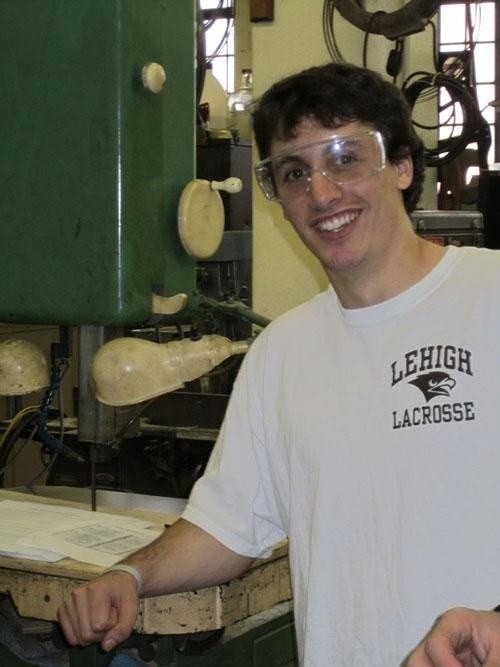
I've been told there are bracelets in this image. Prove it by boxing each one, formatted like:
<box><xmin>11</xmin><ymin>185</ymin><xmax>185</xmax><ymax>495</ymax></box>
<box><xmin>101</xmin><ymin>564</ymin><xmax>143</xmax><ymax>593</ymax></box>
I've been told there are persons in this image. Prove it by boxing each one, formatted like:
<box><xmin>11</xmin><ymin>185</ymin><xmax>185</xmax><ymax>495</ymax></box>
<box><xmin>56</xmin><ymin>61</ymin><xmax>499</xmax><ymax>667</ymax></box>
<box><xmin>400</xmin><ymin>607</ymin><xmax>500</xmax><ymax>667</ymax></box>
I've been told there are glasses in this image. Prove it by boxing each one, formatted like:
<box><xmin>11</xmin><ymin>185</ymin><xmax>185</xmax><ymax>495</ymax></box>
<box><xmin>250</xmin><ymin>127</ymin><xmax>388</xmax><ymax>205</ymax></box>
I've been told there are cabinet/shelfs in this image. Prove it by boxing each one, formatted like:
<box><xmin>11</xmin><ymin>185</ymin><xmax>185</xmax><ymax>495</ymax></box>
<box><xmin>194</xmin><ymin>142</ymin><xmax>255</xmax><ymax>235</ymax></box>
